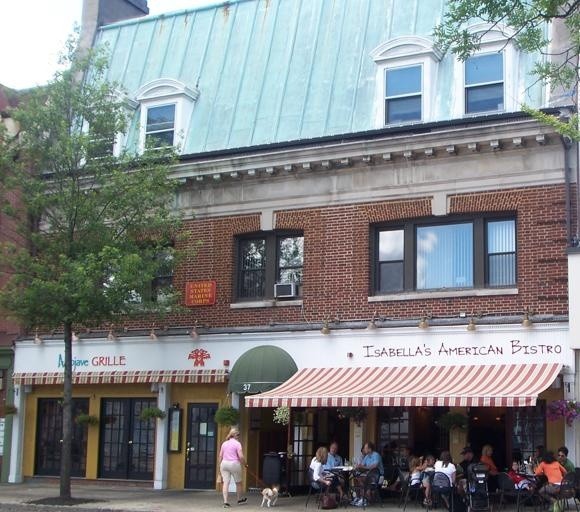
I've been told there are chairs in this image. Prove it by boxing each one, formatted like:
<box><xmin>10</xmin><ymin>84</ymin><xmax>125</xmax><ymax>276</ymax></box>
<box><xmin>303</xmin><ymin>463</ymin><xmax>580</xmax><ymax>511</ymax></box>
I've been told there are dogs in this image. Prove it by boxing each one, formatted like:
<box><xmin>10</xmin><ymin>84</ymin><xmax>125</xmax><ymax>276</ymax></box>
<box><xmin>260</xmin><ymin>483</ymin><xmax>280</xmax><ymax>508</ymax></box>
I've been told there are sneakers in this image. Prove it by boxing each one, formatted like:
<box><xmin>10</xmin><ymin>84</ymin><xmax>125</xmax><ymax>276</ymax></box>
<box><xmin>423</xmin><ymin>498</ymin><xmax>433</xmax><ymax>505</ymax></box>
<box><xmin>224</xmin><ymin>503</ymin><xmax>230</xmax><ymax>507</ymax></box>
<box><xmin>238</xmin><ymin>498</ymin><xmax>247</xmax><ymax>503</ymax></box>
<box><xmin>350</xmin><ymin>497</ymin><xmax>371</xmax><ymax>507</ymax></box>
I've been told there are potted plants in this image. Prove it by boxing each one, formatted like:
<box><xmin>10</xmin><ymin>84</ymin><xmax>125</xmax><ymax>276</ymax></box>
<box><xmin>213</xmin><ymin>407</ymin><xmax>241</xmax><ymax>433</ymax></box>
<box><xmin>139</xmin><ymin>407</ymin><xmax>166</xmax><ymax>428</ymax></box>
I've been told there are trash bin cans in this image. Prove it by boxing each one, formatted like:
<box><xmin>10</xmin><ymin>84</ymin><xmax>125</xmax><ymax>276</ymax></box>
<box><xmin>263</xmin><ymin>454</ymin><xmax>281</xmax><ymax>485</ymax></box>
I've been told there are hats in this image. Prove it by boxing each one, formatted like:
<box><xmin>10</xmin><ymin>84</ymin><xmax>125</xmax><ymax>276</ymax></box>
<box><xmin>460</xmin><ymin>447</ymin><xmax>472</xmax><ymax>455</ymax></box>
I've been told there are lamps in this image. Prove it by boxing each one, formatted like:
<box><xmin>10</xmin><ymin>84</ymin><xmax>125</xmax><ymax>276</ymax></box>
<box><xmin>32</xmin><ymin>319</ymin><xmax>209</xmax><ymax>345</ymax></box>
<box><xmin>320</xmin><ymin>306</ymin><xmax>542</xmax><ymax>335</ymax></box>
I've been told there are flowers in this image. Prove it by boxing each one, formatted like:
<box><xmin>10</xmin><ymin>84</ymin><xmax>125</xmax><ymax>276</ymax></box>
<box><xmin>546</xmin><ymin>397</ymin><xmax>580</xmax><ymax>427</ymax></box>
<box><xmin>273</xmin><ymin>407</ymin><xmax>290</xmax><ymax>427</ymax></box>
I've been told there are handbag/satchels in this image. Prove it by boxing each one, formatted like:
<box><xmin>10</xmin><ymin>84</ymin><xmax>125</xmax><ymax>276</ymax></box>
<box><xmin>320</xmin><ymin>494</ymin><xmax>337</xmax><ymax>509</ymax></box>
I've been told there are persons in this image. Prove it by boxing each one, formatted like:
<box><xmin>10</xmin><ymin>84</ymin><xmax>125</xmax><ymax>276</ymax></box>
<box><xmin>310</xmin><ymin>440</ymin><xmax>576</xmax><ymax>510</ymax></box>
<box><xmin>217</xmin><ymin>426</ymin><xmax>247</xmax><ymax>509</ymax></box>
